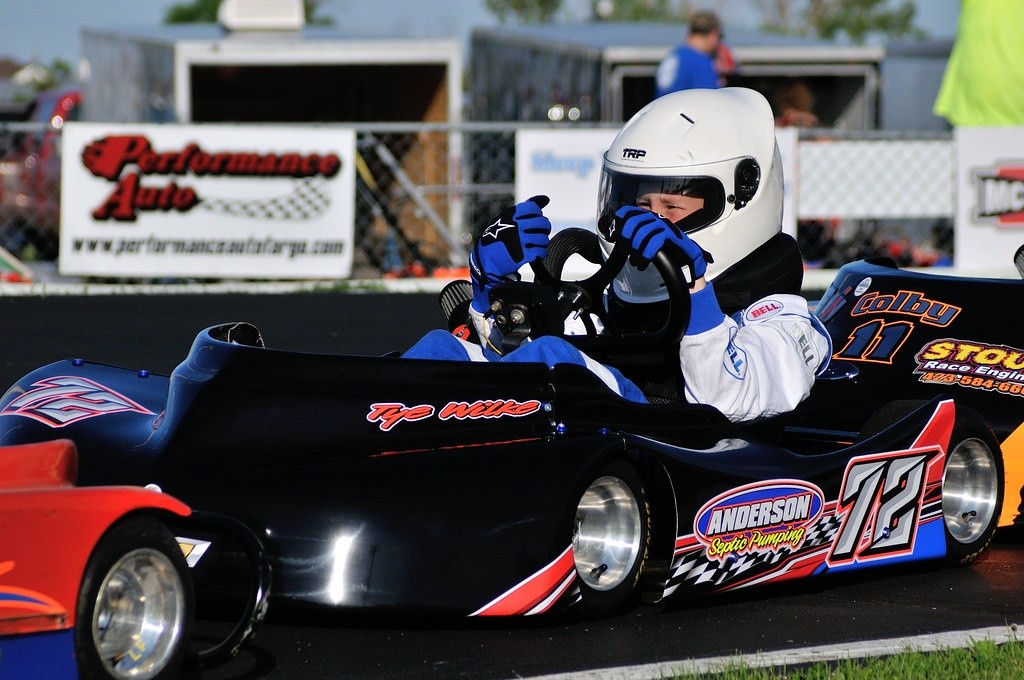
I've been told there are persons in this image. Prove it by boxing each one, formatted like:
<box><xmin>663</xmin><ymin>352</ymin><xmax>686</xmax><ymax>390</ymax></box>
<box><xmin>400</xmin><ymin>85</ymin><xmax>833</xmax><ymax>425</ymax></box>
<box><xmin>770</xmin><ymin>74</ymin><xmax>842</xmax><ymax>266</ymax></box>
<box><xmin>655</xmin><ymin>9</ymin><xmax>725</xmax><ymax>102</ymax></box>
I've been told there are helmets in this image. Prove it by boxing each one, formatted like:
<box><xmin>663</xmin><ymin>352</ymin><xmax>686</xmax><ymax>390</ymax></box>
<box><xmin>595</xmin><ymin>87</ymin><xmax>783</xmax><ymax>306</ymax></box>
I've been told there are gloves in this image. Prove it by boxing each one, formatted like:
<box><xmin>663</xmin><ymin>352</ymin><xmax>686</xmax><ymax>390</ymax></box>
<box><xmin>598</xmin><ymin>206</ymin><xmax>712</xmax><ymax>287</ymax></box>
<box><xmin>467</xmin><ymin>195</ymin><xmax>550</xmax><ymax>312</ymax></box>
